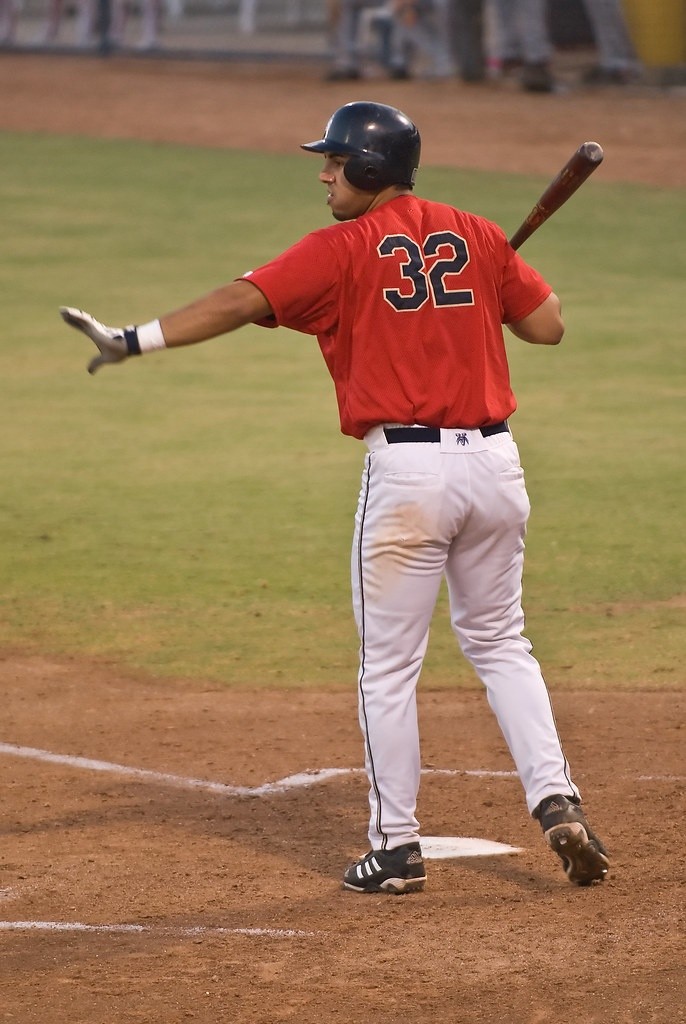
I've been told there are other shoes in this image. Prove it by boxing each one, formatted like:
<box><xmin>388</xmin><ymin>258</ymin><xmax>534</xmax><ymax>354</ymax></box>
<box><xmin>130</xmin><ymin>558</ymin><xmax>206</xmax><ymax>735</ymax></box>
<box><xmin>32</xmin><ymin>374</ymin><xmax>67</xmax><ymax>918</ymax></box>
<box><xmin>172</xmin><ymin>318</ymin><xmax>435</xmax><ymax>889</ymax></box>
<box><xmin>522</xmin><ymin>63</ymin><xmax>553</xmax><ymax>91</ymax></box>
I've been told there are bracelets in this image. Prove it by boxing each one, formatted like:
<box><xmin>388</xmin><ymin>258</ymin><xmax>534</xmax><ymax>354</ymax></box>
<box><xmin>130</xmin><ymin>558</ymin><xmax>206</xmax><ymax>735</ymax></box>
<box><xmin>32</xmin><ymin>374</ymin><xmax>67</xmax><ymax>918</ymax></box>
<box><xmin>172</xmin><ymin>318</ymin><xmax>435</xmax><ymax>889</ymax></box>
<box><xmin>136</xmin><ymin>318</ymin><xmax>166</xmax><ymax>356</ymax></box>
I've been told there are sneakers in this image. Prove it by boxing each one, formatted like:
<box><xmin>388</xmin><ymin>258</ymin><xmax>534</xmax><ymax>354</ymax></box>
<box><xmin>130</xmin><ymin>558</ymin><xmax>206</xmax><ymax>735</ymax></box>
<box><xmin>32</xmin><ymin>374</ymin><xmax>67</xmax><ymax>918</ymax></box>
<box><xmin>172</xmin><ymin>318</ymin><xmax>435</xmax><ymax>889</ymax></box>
<box><xmin>540</xmin><ymin>795</ymin><xmax>610</xmax><ymax>888</ymax></box>
<box><xmin>344</xmin><ymin>841</ymin><xmax>427</xmax><ymax>894</ymax></box>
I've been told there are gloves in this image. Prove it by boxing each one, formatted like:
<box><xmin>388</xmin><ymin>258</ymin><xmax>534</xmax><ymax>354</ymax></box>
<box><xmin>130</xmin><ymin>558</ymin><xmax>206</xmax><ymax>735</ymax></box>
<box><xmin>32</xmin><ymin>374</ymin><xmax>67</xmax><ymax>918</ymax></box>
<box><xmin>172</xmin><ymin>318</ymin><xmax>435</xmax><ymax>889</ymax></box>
<box><xmin>59</xmin><ymin>306</ymin><xmax>166</xmax><ymax>376</ymax></box>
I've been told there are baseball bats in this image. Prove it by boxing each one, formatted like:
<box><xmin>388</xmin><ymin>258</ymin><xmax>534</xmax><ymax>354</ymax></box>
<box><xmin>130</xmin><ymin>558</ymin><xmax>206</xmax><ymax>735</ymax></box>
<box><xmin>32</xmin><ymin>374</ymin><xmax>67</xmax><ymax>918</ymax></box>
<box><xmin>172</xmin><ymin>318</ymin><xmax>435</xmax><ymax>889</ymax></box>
<box><xmin>508</xmin><ymin>139</ymin><xmax>607</xmax><ymax>251</ymax></box>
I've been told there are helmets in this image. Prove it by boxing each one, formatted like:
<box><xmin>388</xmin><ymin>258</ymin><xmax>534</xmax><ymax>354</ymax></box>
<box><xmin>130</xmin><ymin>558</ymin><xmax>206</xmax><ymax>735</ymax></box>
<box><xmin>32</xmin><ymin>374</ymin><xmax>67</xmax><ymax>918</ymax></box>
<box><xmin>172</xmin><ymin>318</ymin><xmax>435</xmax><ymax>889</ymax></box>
<box><xmin>300</xmin><ymin>101</ymin><xmax>421</xmax><ymax>190</ymax></box>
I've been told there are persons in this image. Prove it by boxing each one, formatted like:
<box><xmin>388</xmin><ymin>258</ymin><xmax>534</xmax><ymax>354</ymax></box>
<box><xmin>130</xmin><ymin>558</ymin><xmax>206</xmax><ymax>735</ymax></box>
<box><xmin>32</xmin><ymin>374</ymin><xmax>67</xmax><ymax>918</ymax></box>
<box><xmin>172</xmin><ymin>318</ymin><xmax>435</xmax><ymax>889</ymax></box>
<box><xmin>59</xmin><ymin>100</ymin><xmax>612</xmax><ymax>896</ymax></box>
<box><xmin>322</xmin><ymin>0</ymin><xmax>633</xmax><ymax>102</ymax></box>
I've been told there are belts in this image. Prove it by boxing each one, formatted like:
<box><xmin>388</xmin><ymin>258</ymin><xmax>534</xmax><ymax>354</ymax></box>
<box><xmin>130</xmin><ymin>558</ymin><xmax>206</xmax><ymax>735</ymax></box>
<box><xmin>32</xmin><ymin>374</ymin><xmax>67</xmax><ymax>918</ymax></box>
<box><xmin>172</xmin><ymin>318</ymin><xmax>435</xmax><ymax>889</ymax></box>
<box><xmin>384</xmin><ymin>421</ymin><xmax>510</xmax><ymax>444</ymax></box>
